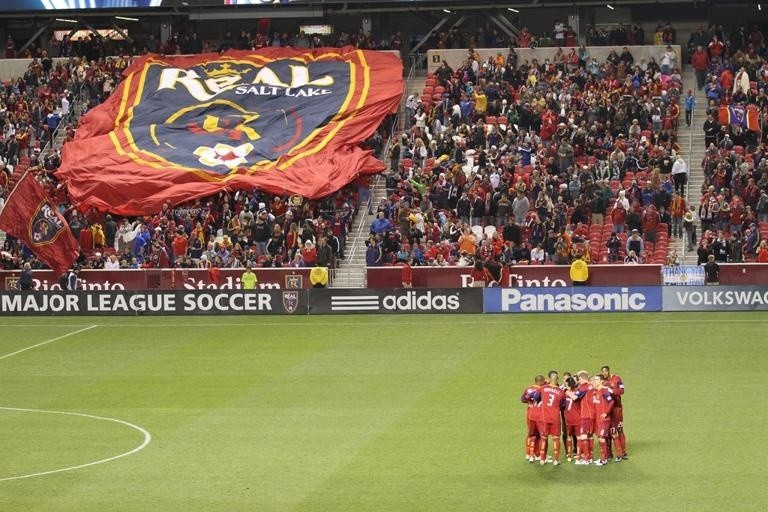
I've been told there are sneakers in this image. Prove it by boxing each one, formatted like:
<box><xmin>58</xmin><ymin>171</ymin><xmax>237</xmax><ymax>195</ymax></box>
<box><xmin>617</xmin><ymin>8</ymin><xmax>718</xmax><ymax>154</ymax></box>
<box><xmin>526</xmin><ymin>454</ymin><xmax>560</xmax><ymax>466</ymax></box>
<box><xmin>594</xmin><ymin>459</ymin><xmax>608</xmax><ymax>466</ymax></box>
<box><xmin>616</xmin><ymin>454</ymin><xmax>628</xmax><ymax>462</ymax></box>
<box><xmin>566</xmin><ymin>453</ymin><xmax>592</xmax><ymax>465</ymax></box>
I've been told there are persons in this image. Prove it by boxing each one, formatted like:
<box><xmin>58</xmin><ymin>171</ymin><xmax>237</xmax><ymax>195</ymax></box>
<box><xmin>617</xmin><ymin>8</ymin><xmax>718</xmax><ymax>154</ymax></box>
<box><xmin>523</xmin><ymin>366</ymin><xmax>628</xmax><ymax>466</ymax></box>
<box><xmin>0</xmin><ymin>22</ymin><xmax>768</xmax><ymax>291</ymax></box>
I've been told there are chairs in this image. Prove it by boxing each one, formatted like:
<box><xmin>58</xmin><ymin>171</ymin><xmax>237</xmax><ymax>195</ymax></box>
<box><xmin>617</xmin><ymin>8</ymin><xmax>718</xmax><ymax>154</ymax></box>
<box><xmin>383</xmin><ymin>71</ymin><xmax>668</xmax><ymax>266</ymax></box>
<box><xmin>703</xmin><ymin>82</ymin><xmax>767</xmax><ymax>263</ymax></box>
<box><xmin>6</xmin><ymin>138</ymin><xmax>41</xmax><ymax>192</ymax></box>
